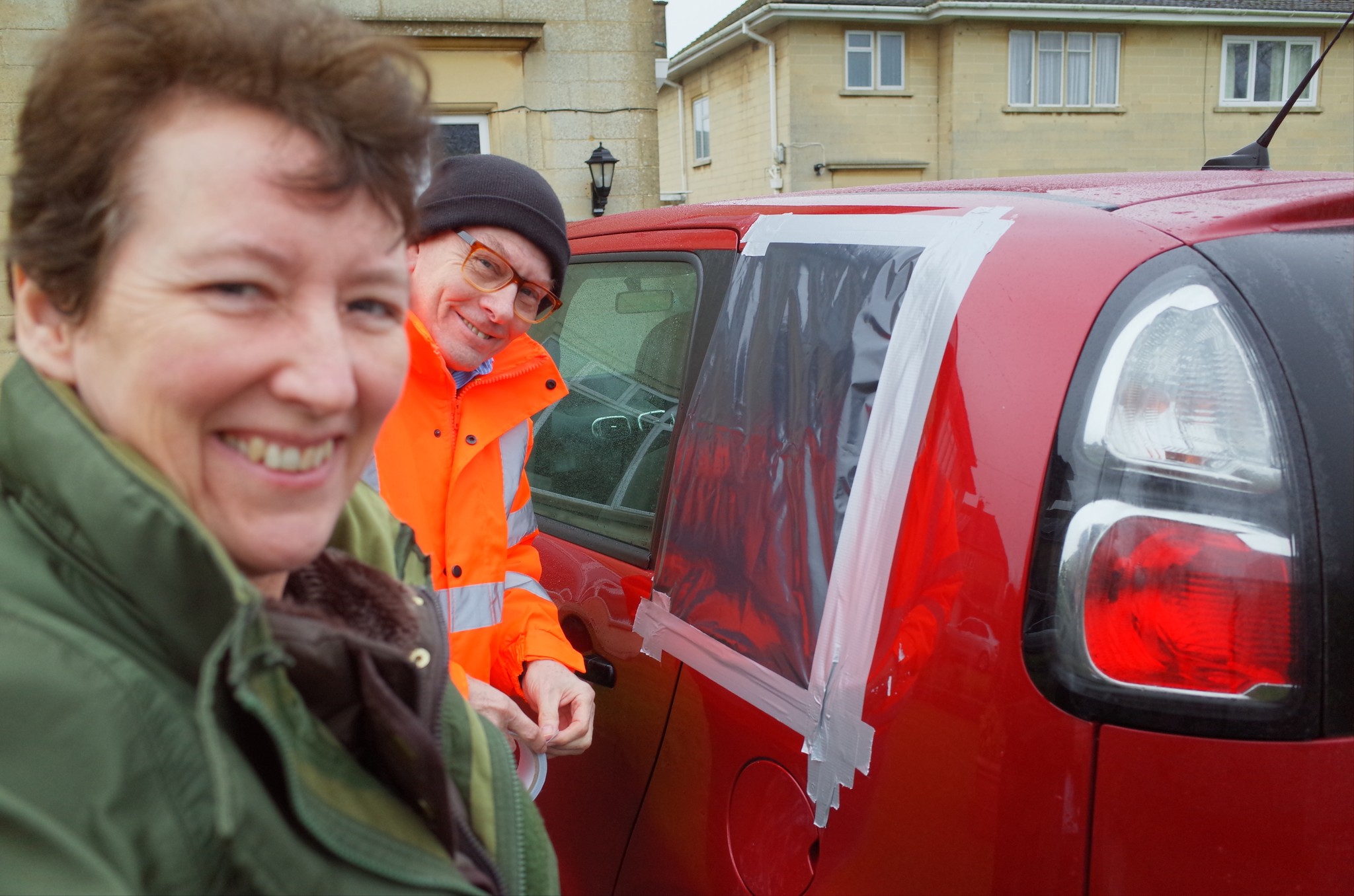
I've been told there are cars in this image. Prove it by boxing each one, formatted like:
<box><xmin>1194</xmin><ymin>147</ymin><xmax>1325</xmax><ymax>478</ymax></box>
<box><xmin>524</xmin><ymin>11</ymin><xmax>1354</xmax><ymax>895</ymax></box>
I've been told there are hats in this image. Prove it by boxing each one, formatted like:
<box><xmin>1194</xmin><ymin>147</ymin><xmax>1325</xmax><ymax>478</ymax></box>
<box><xmin>407</xmin><ymin>154</ymin><xmax>571</xmax><ymax>315</ymax></box>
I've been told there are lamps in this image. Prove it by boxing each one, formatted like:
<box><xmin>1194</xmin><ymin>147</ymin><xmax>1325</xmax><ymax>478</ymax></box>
<box><xmin>583</xmin><ymin>141</ymin><xmax>621</xmax><ymax>218</ymax></box>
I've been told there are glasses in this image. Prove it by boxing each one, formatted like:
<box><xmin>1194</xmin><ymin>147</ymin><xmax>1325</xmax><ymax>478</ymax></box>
<box><xmin>450</xmin><ymin>226</ymin><xmax>563</xmax><ymax>325</ymax></box>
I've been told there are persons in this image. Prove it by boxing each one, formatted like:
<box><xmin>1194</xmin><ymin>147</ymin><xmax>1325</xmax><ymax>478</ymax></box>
<box><xmin>354</xmin><ymin>152</ymin><xmax>598</xmax><ymax>766</ymax></box>
<box><xmin>0</xmin><ymin>0</ymin><xmax>564</xmax><ymax>896</ymax></box>
